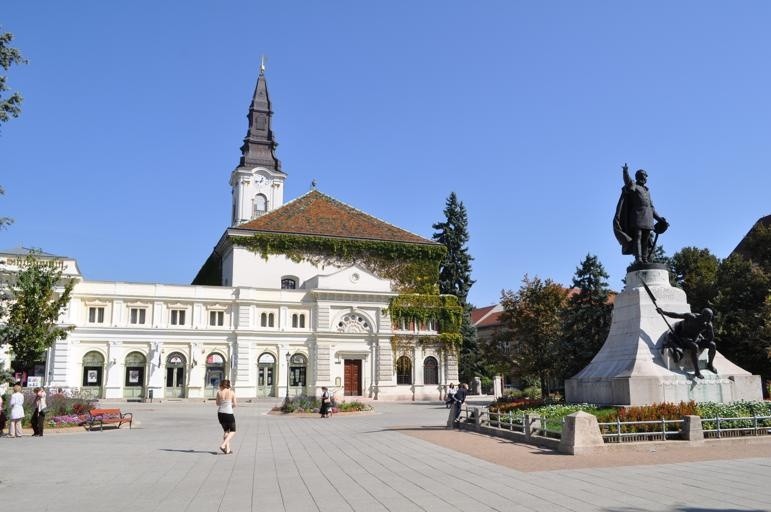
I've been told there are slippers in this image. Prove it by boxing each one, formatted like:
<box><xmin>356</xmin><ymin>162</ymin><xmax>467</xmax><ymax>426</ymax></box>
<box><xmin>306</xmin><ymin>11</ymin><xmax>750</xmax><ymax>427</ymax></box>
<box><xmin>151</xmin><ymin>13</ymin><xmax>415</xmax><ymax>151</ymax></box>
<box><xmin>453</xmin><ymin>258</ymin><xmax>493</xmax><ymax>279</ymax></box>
<box><xmin>220</xmin><ymin>445</ymin><xmax>234</xmax><ymax>454</ymax></box>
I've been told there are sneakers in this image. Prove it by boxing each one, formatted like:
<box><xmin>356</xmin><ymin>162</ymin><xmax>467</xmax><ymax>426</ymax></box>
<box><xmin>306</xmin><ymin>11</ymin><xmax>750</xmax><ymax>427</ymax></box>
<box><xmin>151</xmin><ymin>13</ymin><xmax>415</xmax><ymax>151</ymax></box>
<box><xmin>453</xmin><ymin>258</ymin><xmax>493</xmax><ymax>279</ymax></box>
<box><xmin>446</xmin><ymin>405</ymin><xmax>454</xmax><ymax>408</ymax></box>
<box><xmin>321</xmin><ymin>415</ymin><xmax>330</xmax><ymax>418</ymax></box>
<box><xmin>454</xmin><ymin>418</ymin><xmax>461</xmax><ymax>423</ymax></box>
<box><xmin>0</xmin><ymin>431</ymin><xmax>44</xmax><ymax>438</ymax></box>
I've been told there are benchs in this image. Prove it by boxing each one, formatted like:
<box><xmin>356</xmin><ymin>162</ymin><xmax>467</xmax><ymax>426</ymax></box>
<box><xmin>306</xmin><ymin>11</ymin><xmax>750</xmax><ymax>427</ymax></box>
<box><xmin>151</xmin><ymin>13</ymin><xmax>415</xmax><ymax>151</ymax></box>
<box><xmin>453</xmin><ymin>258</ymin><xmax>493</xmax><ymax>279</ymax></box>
<box><xmin>88</xmin><ymin>407</ymin><xmax>133</xmax><ymax>432</ymax></box>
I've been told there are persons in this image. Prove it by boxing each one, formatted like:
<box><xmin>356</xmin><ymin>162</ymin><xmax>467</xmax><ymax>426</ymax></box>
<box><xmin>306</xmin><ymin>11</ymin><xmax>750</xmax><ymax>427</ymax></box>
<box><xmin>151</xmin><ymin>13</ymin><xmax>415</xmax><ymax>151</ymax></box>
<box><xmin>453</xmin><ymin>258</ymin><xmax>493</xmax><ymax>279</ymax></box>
<box><xmin>656</xmin><ymin>307</ymin><xmax>718</xmax><ymax>379</ymax></box>
<box><xmin>0</xmin><ymin>397</ymin><xmax>7</xmax><ymax>436</ymax></box>
<box><xmin>320</xmin><ymin>386</ymin><xmax>338</xmax><ymax>417</ymax></box>
<box><xmin>445</xmin><ymin>383</ymin><xmax>455</xmax><ymax>408</ymax></box>
<box><xmin>6</xmin><ymin>385</ymin><xmax>24</xmax><ymax>438</ymax></box>
<box><xmin>31</xmin><ymin>387</ymin><xmax>47</xmax><ymax>438</ymax></box>
<box><xmin>54</xmin><ymin>388</ymin><xmax>65</xmax><ymax>396</ymax></box>
<box><xmin>612</xmin><ymin>162</ymin><xmax>670</xmax><ymax>263</ymax></box>
<box><xmin>454</xmin><ymin>383</ymin><xmax>469</xmax><ymax>423</ymax></box>
<box><xmin>216</xmin><ymin>379</ymin><xmax>237</xmax><ymax>454</ymax></box>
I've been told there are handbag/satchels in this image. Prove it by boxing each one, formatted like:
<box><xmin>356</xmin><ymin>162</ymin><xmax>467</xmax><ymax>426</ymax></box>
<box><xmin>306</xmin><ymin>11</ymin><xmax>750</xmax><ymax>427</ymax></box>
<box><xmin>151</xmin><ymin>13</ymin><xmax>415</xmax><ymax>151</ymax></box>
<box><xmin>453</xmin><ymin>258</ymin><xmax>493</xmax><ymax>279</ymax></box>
<box><xmin>325</xmin><ymin>402</ymin><xmax>334</xmax><ymax>407</ymax></box>
